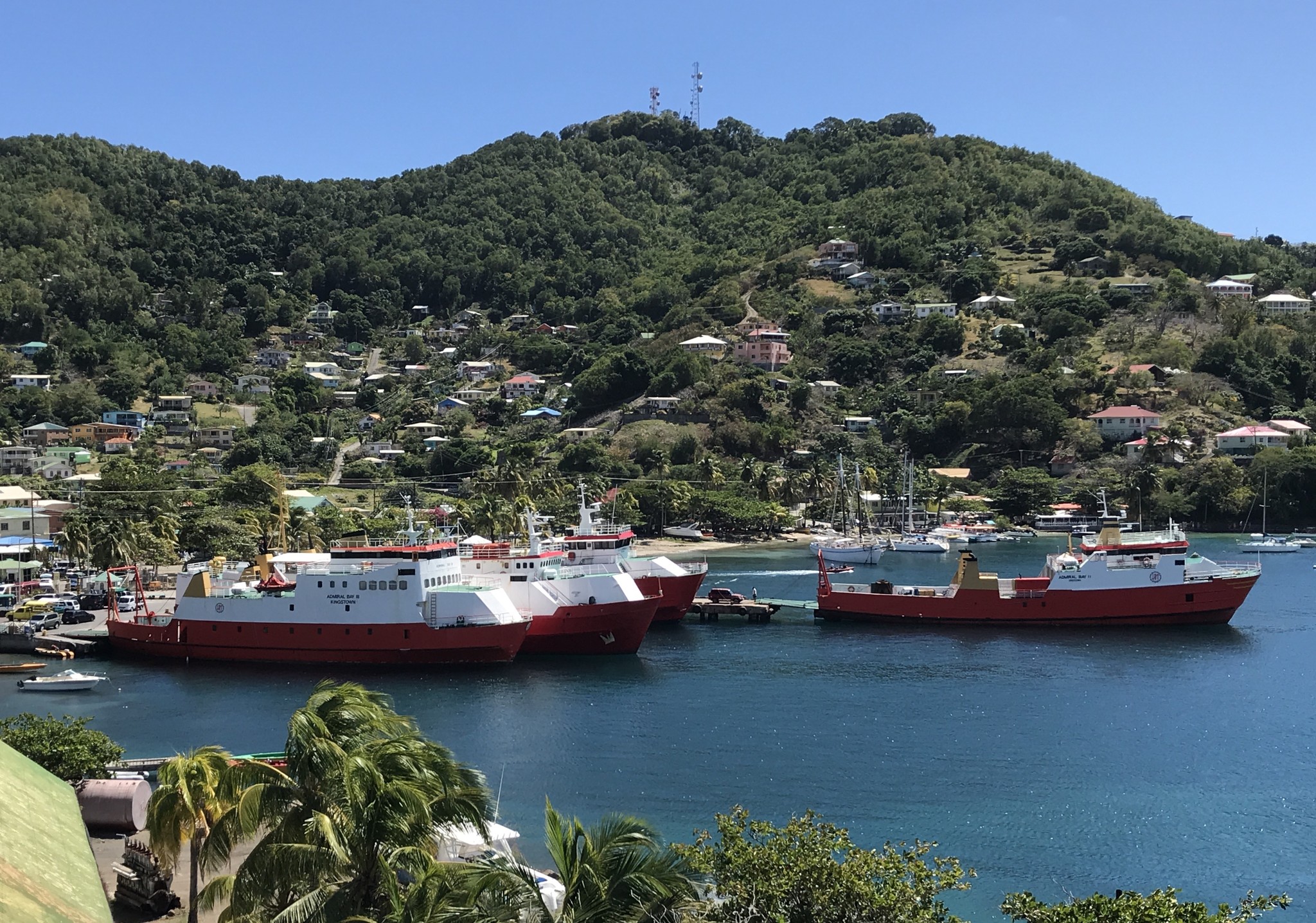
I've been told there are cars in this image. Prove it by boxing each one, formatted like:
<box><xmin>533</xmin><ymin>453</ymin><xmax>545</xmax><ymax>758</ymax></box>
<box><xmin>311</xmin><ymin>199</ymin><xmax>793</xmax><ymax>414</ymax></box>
<box><xmin>0</xmin><ymin>558</ymin><xmax>130</xmax><ymax>618</ymax></box>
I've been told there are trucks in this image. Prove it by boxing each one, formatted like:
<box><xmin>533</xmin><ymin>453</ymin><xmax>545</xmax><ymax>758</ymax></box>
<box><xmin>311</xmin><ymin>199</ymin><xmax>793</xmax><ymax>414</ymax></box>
<box><xmin>117</xmin><ymin>595</ymin><xmax>144</xmax><ymax>612</ymax></box>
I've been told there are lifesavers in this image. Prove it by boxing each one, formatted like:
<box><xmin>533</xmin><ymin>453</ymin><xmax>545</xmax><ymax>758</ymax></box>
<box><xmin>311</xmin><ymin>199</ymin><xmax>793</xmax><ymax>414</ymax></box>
<box><xmin>848</xmin><ymin>586</ymin><xmax>855</xmax><ymax>592</ymax></box>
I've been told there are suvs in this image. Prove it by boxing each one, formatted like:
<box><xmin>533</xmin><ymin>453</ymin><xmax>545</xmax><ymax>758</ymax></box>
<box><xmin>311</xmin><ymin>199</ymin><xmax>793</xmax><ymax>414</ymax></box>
<box><xmin>29</xmin><ymin>612</ymin><xmax>61</xmax><ymax>632</ymax></box>
<box><xmin>708</xmin><ymin>588</ymin><xmax>744</xmax><ymax>604</ymax></box>
<box><xmin>54</xmin><ymin>599</ymin><xmax>80</xmax><ymax>614</ymax></box>
<box><xmin>61</xmin><ymin>610</ymin><xmax>95</xmax><ymax>624</ymax></box>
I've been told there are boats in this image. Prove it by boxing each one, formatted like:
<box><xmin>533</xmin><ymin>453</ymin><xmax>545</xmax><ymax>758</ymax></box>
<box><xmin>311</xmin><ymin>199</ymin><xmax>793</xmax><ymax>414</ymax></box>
<box><xmin>253</xmin><ymin>574</ymin><xmax>296</xmax><ymax>593</ymax></box>
<box><xmin>226</xmin><ymin>751</ymin><xmax>289</xmax><ymax>776</ymax></box>
<box><xmin>0</xmin><ymin>663</ymin><xmax>48</xmax><ymax>673</ymax></box>
<box><xmin>1235</xmin><ymin>467</ymin><xmax>1316</xmax><ymax>551</ymax></box>
<box><xmin>662</xmin><ymin>521</ymin><xmax>718</xmax><ymax>540</ymax></box>
<box><xmin>104</xmin><ymin>473</ymin><xmax>709</xmax><ymax>668</ymax></box>
<box><xmin>1070</xmin><ymin>488</ymin><xmax>1190</xmax><ymax>556</ymax></box>
<box><xmin>433</xmin><ymin>762</ymin><xmax>566</xmax><ymax>923</ymax></box>
<box><xmin>813</xmin><ymin>547</ymin><xmax>1263</xmax><ymax>626</ymax></box>
<box><xmin>17</xmin><ymin>669</ymin><xmax>106</xmax><ymax>691</ymax></box>
<box><xmin>809</xmin><ymin>448</ymin><xmax>1038</xmax><ymax>564</ymax></box>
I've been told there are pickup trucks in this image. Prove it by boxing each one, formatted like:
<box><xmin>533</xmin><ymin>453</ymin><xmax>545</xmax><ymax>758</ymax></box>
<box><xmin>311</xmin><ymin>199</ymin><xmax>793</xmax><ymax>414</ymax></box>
<box><xmin>5</xmin><ymin>606</ymin><xmax>56</xmax><ymax>621</ymax></box>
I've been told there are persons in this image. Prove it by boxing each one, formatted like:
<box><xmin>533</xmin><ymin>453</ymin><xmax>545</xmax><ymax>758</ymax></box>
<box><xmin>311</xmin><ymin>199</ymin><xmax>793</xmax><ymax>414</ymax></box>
<box><xmin>830</xmin><ymin>561</ymin><xmax>834</xmax><ymax>569</ymax></box>
<box><xmin>752</xmin><ymin>586</ymin><xmax>757</xmax><ymax>602</ymax></box>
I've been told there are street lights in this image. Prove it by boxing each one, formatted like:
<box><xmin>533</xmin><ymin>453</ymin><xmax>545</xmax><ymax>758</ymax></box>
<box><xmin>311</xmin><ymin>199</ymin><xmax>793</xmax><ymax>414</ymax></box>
<box><xmin>19</xmin><ymin>533</ymin><xmax>41</xmax><ymax>605</ymax></box>
